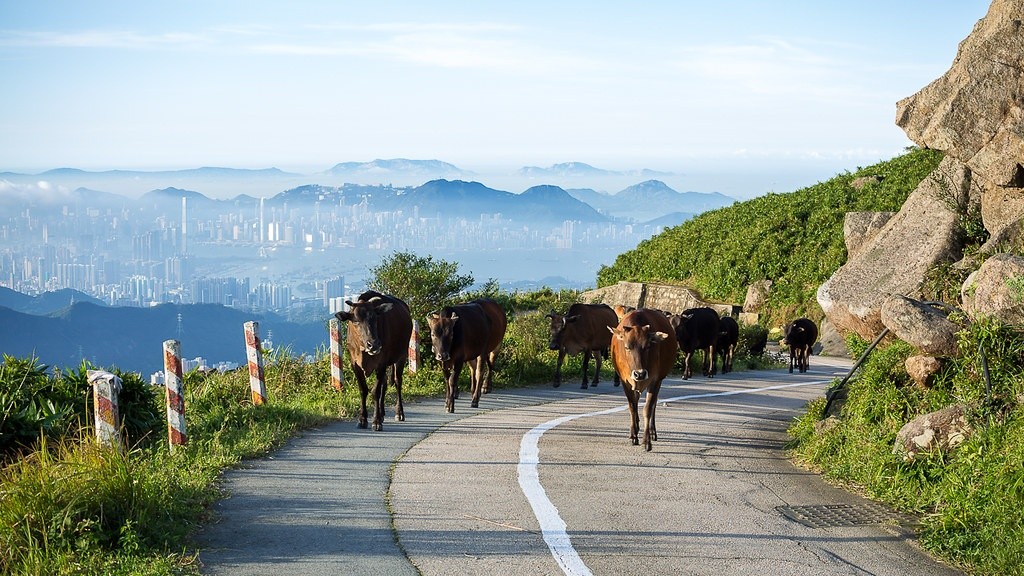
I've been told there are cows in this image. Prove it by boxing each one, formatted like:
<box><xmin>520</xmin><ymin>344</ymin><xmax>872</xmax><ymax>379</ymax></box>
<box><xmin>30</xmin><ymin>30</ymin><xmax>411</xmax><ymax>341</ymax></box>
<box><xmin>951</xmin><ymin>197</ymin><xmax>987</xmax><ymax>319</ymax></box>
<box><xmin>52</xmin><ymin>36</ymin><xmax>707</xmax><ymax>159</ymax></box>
<box><xmin>663</xmin><ymin>307</ymin><xmax>739</xmax><ymax>381</ymax></box>
<box><xmin>783</xmin><ymin>318</ymin><xmax>819</xmax><ymax>374</ymax></box>
<box><xmin>544</xmin><ymin>303</ymin><xmax>619</xmax><ymax>389</ymax></box>
<box><xmin>425</xmin><ymin>298</ymin><xmax>507</xmax><ymax>413</ymax></box>
<box><xmin>334</xmin><ymin>291</ymin><xmax>413</xmax><ymax>431</ymax></box>
<box><xmin>611</xmin><ymin>308</ymin><xmax>677</xmax><ymax>451</ymax></box>
